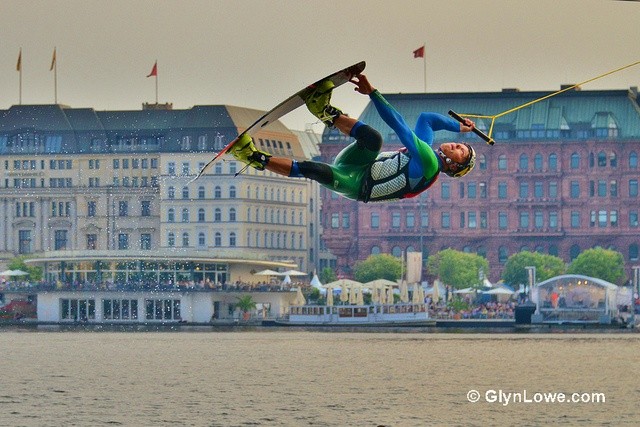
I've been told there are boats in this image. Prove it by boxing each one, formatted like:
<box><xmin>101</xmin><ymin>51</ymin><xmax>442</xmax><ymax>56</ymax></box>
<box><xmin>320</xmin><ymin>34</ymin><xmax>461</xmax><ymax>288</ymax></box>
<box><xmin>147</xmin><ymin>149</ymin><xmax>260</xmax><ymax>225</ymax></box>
<box><xmin>274</xmin><ymin>302</ymin><xmax>437</xmax><ymax>326</ymax></box>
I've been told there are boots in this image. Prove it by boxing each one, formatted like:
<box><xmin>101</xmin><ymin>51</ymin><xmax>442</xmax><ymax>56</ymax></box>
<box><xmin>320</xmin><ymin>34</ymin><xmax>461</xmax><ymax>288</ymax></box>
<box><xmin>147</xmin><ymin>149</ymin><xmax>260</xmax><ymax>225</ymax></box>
<box><xmin>305</xmin><ymin>80</ymin><xmax>348</xmax><ymax>130</ymax></box>
<box><xmin>231</xmin><ymin>134</ymin><xmax>271</xmax><ymax>171</ymax></box>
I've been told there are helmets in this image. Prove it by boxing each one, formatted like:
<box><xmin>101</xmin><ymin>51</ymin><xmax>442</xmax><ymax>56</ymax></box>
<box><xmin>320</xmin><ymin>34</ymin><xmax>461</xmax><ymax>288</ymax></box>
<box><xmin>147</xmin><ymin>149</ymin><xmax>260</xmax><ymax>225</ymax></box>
<box><xmin>447</xmin><ymin>142</ymin><xmax>476</xmax><ymax>178</ymax></box>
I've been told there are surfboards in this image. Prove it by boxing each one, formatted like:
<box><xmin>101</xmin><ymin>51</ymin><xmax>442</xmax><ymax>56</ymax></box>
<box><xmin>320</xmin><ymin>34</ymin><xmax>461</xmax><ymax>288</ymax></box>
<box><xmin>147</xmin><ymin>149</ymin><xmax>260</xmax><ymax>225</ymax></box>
<box><xmin>193</xmin><ymin>61</ymin><xmax>366</xmax><ymax>180</ymax></box>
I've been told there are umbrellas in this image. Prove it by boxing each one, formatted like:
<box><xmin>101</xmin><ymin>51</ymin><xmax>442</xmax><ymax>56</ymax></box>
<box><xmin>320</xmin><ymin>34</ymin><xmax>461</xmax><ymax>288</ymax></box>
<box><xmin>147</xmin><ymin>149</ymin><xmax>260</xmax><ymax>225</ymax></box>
<box><xmin>1</xmin><ymin>268</ymin><xmax>30</xmax><ymax>276</ymax></box>
<box><xmin>279</xmin><ymin>269</ymin><xmax>309</xmax><ymax>276</ymax></box>
<box><xmin>254</xmin><ymin>268</ymin><xmax>283</xmax><ymax>276</ymax></box>
<box><xmin>290</xmin><ymin>277</ymin><xmax>440</xmax><ymax>305</ymax></box>
<box><xmin>481</xmin><ymin>286</ymin><xmax>516</xmax><ymax>295</ymax></box>
<box><xmin>452</xmin><ymin>285</ymin><xmax>487</xmax><ymax>294</ymax></box>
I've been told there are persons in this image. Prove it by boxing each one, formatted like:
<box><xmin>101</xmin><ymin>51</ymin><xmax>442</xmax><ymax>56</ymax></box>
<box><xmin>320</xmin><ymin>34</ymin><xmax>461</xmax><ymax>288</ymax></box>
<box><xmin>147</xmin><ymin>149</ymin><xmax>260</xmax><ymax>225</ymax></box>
<box><xmin>225</xmin><ymin>71</ymin><xmax>477</xmax><ymax>204</ymax></box>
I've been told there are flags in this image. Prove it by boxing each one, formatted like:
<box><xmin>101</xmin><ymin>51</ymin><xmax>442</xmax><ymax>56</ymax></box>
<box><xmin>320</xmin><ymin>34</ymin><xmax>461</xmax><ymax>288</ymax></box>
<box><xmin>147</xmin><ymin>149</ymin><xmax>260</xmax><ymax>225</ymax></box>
<box><xmin>147</xmin><ymin>59</ymin><xmax>158</xmax><ymax>78</ymax></box>
<box><xmin>50</xmin><ymin>46</ymin><xmax>57</xmax><ymax>71</ymax></box>
<box><xmin>414</xmin><ymin>43</ymin><xmax>426</xmax><ymax>58</ymax></box>
<box><xmin>16</xmin><ymin>45</ymin><xmax>22</xmax><ymax>71</ymax></box>
